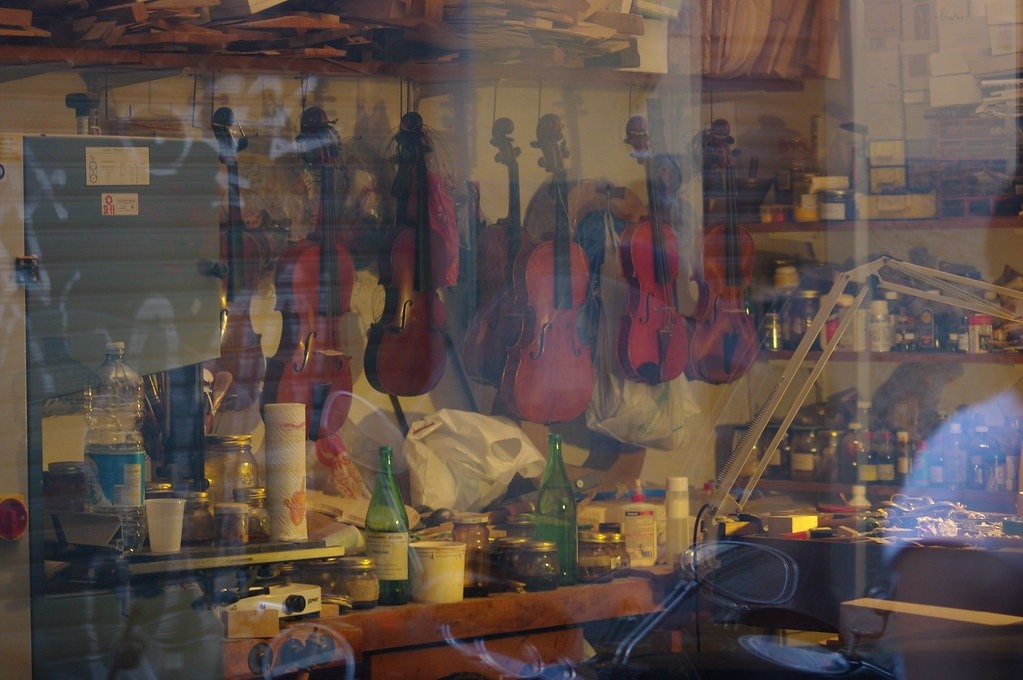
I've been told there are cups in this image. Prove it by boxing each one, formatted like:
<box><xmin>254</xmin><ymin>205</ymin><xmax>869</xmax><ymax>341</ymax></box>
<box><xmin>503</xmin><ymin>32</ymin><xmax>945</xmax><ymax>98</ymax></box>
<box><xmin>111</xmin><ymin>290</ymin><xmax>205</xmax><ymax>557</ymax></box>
<box><xmin>410</xmin><ymin>541</ymin><xmax>467</xmax><ymax>602</ymax></box>
<box><xmin>144</xmin><ymin>498</ymin><xmax>187</xmax><ymax>553</ymax></box>
<box><xmin>43</xmin><ymin>461</ymin><xmax>84</xmax><ymax>504</ymax></box>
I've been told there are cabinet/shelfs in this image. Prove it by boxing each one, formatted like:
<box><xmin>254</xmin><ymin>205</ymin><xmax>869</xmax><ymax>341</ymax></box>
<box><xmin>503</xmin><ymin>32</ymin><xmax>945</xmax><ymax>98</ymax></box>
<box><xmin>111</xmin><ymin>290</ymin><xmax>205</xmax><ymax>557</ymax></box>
<box><xmin>717</xmin><ymin>215</ymin><xmax>1023</xmax><ymax>515</ymax></box>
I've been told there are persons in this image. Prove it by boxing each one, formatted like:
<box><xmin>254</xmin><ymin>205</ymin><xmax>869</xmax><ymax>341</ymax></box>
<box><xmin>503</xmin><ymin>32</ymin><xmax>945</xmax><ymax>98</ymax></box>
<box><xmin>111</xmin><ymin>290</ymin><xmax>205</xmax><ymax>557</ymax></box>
<box><xmin>875</xmin><ymin>157</ymin><xmax>1022</xmax><ymax>680</ymax></box>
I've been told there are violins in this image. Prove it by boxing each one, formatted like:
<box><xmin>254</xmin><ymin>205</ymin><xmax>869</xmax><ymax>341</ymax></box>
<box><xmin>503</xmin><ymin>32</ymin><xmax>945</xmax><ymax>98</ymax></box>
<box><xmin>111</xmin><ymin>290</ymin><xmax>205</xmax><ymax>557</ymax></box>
<box><xmin>364</xmin><ymin>110</ymin><xmax>450</xmax><ymax>399</ymax></box>
<box><xmin>461</xmin><ymin>117</ymin><xmax>533</xmax><ymax>389</ymax></box>
<box><xmin>496</xmin><ymin>114</ymin><xmax>598</xmax><ymax>424</ymax></box>
<box><xmin>685</xmin><ymin>118</ymin><xmax>758</xmax><ymax>386</ymax></box>
<box><xmin>616</xmin><ymin>114</ymin><xmax>691</xmax><ymax>385</ymax></box>
<box><xmin>259</xmin><ymin>104</ymin><xmax>356</xmax><ymax>442</ymax></box>
<box><xmin>190</xmin><ymin>102</ymin><xmax>273</xmax><ymax>413</ymax></box>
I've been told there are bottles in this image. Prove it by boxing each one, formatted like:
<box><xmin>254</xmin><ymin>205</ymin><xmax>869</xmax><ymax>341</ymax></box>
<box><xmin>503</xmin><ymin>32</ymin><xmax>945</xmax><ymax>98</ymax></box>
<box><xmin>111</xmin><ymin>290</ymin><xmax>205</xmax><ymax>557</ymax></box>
<box><xmin>84</xmin><ymin>343</ymin><xmax>144</xmax><ymax>551</ymax></box>
<box><xmin>576</xmin><ymin>482</ymin><xmax>657</xmax><ymax>582</ymax></box>
<box><xmin>452</xmin><ymin>511</ymin><xmax>559</xmax><ymax>596</ymax></box>
<box><xmin>665</xmin><ymin>476</ymin><xmax>688</xmax><ymax>559</ymax></box>
<box><xmin>181</xmin><ymin>430</ymin><xmax>269</xmax><ymax>546</ymax></box>
<box><xmin>845</xmin><ymin>484</ymin><xmax>872</xmax><ymax>507</ymax></box>
<box><xmin>535</xmin><ymin>436</ymin><xmax>577</xmax><ymax>585</ymax></box>
<box><xmin>743</xmin><ymin>133</ymin><xmax>855</xmax><ymax>220</ymax></box>
<box><xmin>761</xmin><ymin>265</ymin><xmax>969</xmax><ymax>353</ymax></box>
<box><xmin>929</xmin><ymin>402</ymin><xmax>1023</xmax><ymax>489</ymax></box>
<box><xmin>279</xmin><ymin>448</ymin><xmax>409</xmax><ymax>610</ymax></box>
<box><xmin>734</xmin><ymin>401</ymin><xmax>929</xmax><ymax>487</ymax></box>
